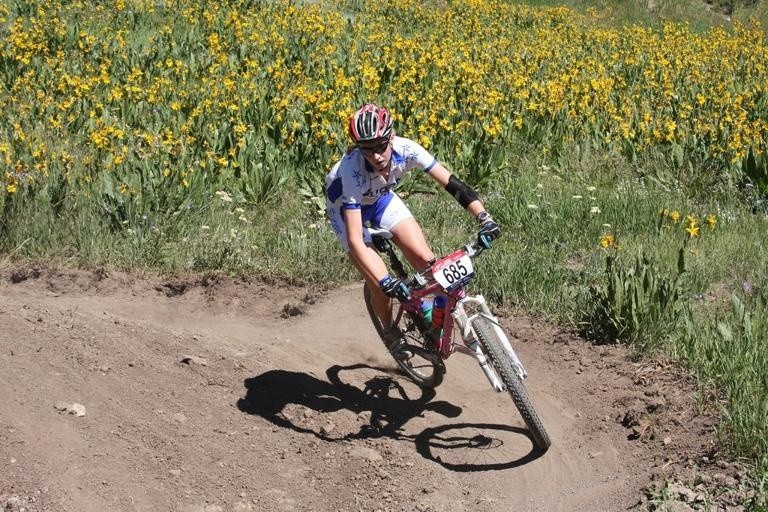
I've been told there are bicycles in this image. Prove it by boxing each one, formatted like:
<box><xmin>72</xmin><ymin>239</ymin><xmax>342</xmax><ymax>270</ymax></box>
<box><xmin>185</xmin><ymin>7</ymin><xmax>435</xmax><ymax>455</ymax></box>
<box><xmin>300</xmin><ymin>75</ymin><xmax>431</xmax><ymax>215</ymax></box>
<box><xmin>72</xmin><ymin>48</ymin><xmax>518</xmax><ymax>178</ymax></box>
<box><xmin>362</xmin><ymin>227</ymin><xmax>551</xmax><ymax>450</ymax></box>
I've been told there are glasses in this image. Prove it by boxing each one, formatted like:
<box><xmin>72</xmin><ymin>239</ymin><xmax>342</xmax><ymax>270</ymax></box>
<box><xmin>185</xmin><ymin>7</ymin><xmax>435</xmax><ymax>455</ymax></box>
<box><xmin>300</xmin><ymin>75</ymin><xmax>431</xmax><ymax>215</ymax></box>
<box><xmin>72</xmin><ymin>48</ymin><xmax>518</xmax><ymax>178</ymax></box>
<box><xmin>357</xmin><ymin>137</ymin><xmax>391</xmax><ymax>157</ymax></box>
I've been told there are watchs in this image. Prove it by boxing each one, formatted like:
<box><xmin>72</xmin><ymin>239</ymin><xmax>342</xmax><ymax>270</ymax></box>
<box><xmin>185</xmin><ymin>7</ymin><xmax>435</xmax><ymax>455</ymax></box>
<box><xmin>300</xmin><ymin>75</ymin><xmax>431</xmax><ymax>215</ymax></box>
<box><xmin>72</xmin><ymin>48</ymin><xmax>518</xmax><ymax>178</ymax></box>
<box><xmin>477</xmin><ymin>211</ymin><xmax>490</xmax><ymax>223</ymax></box>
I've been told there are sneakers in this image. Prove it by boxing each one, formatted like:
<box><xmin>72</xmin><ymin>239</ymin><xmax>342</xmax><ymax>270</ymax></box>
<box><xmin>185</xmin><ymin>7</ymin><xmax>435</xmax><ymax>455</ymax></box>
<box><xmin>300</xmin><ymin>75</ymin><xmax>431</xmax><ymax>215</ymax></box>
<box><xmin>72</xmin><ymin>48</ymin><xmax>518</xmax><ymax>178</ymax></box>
<box><xmin>383</xmin><ymin>320</ymin><xmax>413</xmax><ymax>360</ymax></box>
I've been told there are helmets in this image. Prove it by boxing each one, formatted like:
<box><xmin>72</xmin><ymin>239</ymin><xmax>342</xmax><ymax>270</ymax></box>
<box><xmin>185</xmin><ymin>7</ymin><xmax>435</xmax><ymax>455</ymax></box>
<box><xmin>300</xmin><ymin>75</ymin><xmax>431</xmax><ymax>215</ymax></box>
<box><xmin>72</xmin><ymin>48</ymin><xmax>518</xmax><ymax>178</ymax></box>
<box><xmin>349</xmin><ymin>103</ymin><xmax>394</xmax><ymax>144</ymax></box>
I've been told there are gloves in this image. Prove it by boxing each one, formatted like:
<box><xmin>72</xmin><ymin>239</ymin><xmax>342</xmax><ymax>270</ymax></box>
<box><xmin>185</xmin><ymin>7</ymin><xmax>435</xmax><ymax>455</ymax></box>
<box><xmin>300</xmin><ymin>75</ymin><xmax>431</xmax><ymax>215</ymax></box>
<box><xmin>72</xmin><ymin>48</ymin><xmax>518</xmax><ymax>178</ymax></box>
<box><xmin>380</xmin><ymin>274</ymin><xmax>410</xmax><ymax>303</ymax></box>
<box><xmin>476</xmin><ymin>211</ymin><xmax>502</xmax><ymax>249</ymax></box>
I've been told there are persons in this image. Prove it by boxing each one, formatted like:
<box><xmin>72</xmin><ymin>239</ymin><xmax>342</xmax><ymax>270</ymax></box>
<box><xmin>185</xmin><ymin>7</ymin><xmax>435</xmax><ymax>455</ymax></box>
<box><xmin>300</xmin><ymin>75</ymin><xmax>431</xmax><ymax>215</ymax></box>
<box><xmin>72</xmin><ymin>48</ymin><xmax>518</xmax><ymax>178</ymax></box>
<box><xmin>325</xmin><ymin>103</ymin><xmax>502</xmax><ymax>360</ymax></box>
